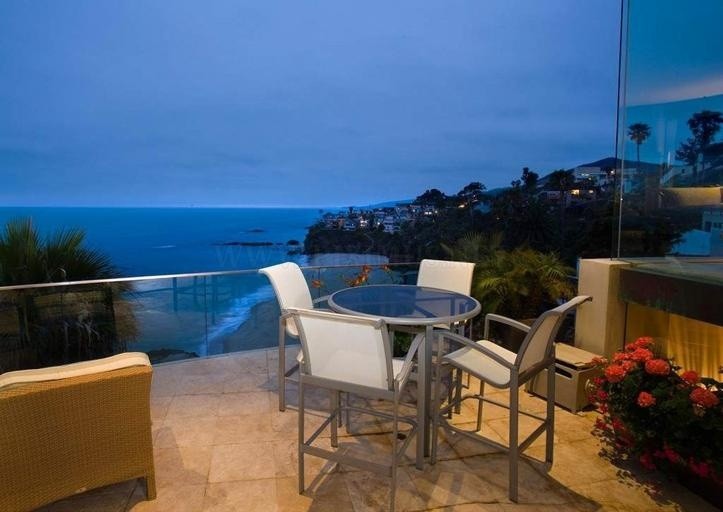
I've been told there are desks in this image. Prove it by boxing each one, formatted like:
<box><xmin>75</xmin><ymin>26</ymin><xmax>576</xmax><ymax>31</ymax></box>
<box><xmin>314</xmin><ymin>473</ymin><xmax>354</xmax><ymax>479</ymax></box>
<box><xmin>328</xmin><ymin>283</ymin><xmax>484</xmax><ymax>463</ymax></box>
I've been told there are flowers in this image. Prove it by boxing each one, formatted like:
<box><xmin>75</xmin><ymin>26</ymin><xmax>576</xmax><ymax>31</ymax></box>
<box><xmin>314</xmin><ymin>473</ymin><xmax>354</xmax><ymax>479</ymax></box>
<box><xmin>584</xmin><ymin>335</ymin><xmax>723</xmax><ymax>496</ymax></box>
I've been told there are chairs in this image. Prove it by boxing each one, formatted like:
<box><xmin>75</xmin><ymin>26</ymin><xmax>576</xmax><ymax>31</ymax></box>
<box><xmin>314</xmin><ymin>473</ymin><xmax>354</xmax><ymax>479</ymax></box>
<box><xmin>397</xmin><ymin>258</ymin><xmax>478</xmax><ymax>418</ymax></box>
<box><xmin>258</xmin><ymin>260</ymin><xmax>351</xmax><ymax>434</ymax></box>
<box><xmin>434</xmin><ymin>292</ymin><xmax>591</xmax><ymax>501</ymax></box>
<box><xmin>287</xmin><ymin>306</ymin><xmax>431</xmax><ymax>509</ymax></box>
<box><xmin>0</xmin><ymin>351</ymin><xmax>157</xmax><ymax>512</ymax></box>
